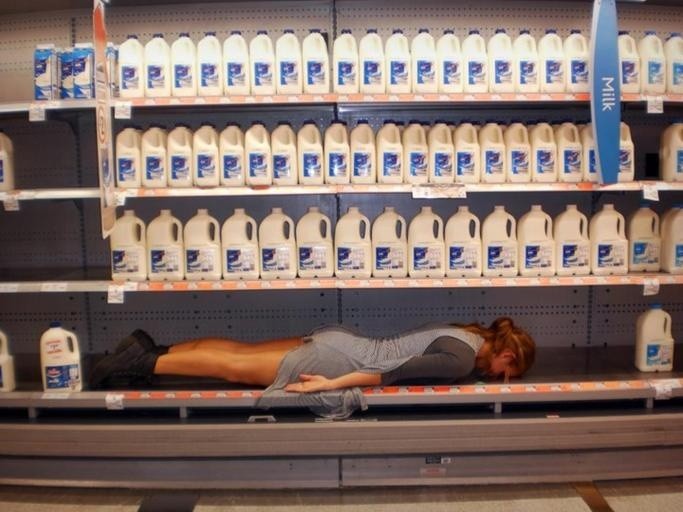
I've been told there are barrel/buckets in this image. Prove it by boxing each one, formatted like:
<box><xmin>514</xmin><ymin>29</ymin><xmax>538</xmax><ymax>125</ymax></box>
<box><xmin>146</xmin><ymin>211</ymin><xmax>183</xmax><ymax>280</ymax></box>
<box><xmin>221</xmin><ymin>208</ymin><xmax>259</xmax><ymax>280</ymax></box>
<box><xmin>302</xmin><ymin>29</ymin><xmax>330</xmax><ymax>93</ymax></box>
<box><xmin>192</xmin><ymin>122</ymin><xmax>219</xmax><ymax>186</ymax></box>
<box><xmin>526</xmin><ymin>118</ymin><xmax>537</xmax><ymax>132</ymax></box>
<box><xmin>272</xmin><ymin>121</ymin><xmax>298</xmax><ymax>186</ymax></box>
<box><xmin>626</xmin><ymin>202</ymin><xmax>661</xmax><ymax>272</ymax></box>
<box><xmin>530</xmin><ymin>119</ymin><xmax>556</xmax><ymax>183</ymax></box>
<box><xmin>664</xmin><ymin>32</ymin><xmax>683</xmax><ymax>95</ymax></box>
<box><xmin>0</xmin><ymin>128</ymin><xmax>15</xmax><ymax>196</ymax></box>
<box><xmin>444</xmin><ymin>206</ymin><xmax>482</xmax><ymax>275</ymax></box>
<box><xmin>462</xmin><ymin>30</ymin><xmax>489</xmax><ymax>93</ymax></box>
<box><xmin>385</xmin><ymin>30</ymin><xmax>411</xmax><ymax>93</ymax></box>
<box><xmin>555</xmin><ymin>120</ymin><xmax>583</xmax><ymax>183</ymax></box>
<box><xmin>0</xmin><ymin>331</ymin><xmax>16</xmax><ymax>392</ymax></box>
<box><xmin>224</xmin><ymin>31</ymin><xmax>251</xmax><ymax>95</ymax></box>
<box><xmin>349</xmin><ymin>120</ymin><xmax>377</xmax><ymax>183</ymax></box>
<box><xmin>275</xmin><ymin>30</ymin><xmax>302</xmax><ymax>94</ymax></box>
<box><xmin>638</xmin><ymin>30</ymin><xmax>667</xmax><ymax>93</ymax></box>
<box><xmin>617</xmin><ymin>30</ymin><xmax>640</xmax><ymax>92</ymax></box>
<box><xmin>512</xmin><ymin>30</ymin><xmax>540</xmax><ymax>93</ymax></box>
<box><xmin>372</xmin><ymin>206</ymin><xmax>408</xmax><ymax>278</ymax></box>
<box><xmin>473</xmin><ymin>120</ymin><xmax>483</xmax><ymax>131</ymax></box>
<box><xmin>481</xmin><ymin>204</ymin><xmax>518</xmax><ymax>276</ymax></box>
<box><xmin>396</xmin><ymin>121</ymin><xmax>405</xmax><ymax>135</ymax></box>
<box><xmin>401</xmin><ymin>120</ymin><xmax>428</xmax><ymax>185</ymax></box>
<box><xmin>167</xmin><ymin>123</ymin><xmax>192</xmax><ymax>187</ymax></box>
<box><xmin>358</xmin><ymin>29</ymin><xmax>386</xmax><ymax>93</ymax></box>
<box><xmin>582</xmin><ymin>119</ymin><xmax>599</xmax><ymax>183</ymax></box>
<box><xmin>588</xmin><ymin>202</ymin><xmax>628</xmax><ymax>274</ymax></box>
<box><xmin>410</xmin><ymin>28</ymin><xmax>438</xmax><ymax>93</ymax></box>
<box><xmin>296</xmin><ymin>121</ymin><xmax>324</xmax><ymax>185</ymax></box>
<box><xmin>110</xmin><ymin>210</ymin><xmax>147</xmax><ymax>281</ymax></box>
<box><xmin>258</xmin><ymin>208</ymin><xmax>297</xmax><ymax>278</ymax></box>
<box><xmin>332</xmin><ymin>30</ymin><xmax>359</xmax><ymax>93</ymax></box>
<box><xmin>575</xmin><ymin>120</ymin><xmax>587</xmax><ymax>132</ymax></box>
<box><xmin>407</xmin><ymin>206</ymin><xmax>445</xmax><ymax>276</ymax></box>
<box><xmin>518</xmin><ymin>204</ymin><xmax>555</xmax><ymax>276</ymax></box>
<box><xmin>142</xmin><ymin>33</ymin><xmax>172</xmax><ymax>97</ymax></box>
<box><xmin>503</xmin><ymin>119</ymin><xmax>532</xmax><ymax>183</ymax></box>
<box><xmin>618</xmin><ymin>121</ymin><xmax>635</xmax><ymax>181</ymax></box>
<box><xmin>249</xmin><ymin>30</ymin><xmax>276</xmax><ymax>96</ymax></box>
<box><xmin>422</xmin><ymin>120</ymin><xmax>432</xmax><ymax>133</ymax></box>
<box><xmin>118</xmin><ymin>34</ymin><xmax>143</xmax><ymax>97</ymax></box>
<box><xmin>141</xmin><ymin>122</ymin><xmax>167</xmax><ymax>188</ymax></box>
<box><xmin>245</xmin><ymin>120</ymin><xmax>271</xmax><ymax>186</ymax></box>
<box><xmin>435</xmin><ymin>30</ymin><xmax>463</xmax><ymax>93</ymax></box>
<box><xmin>40</xmin><ymin>323</ymin><xmax>83</xmax><ymax>392</ymax></box>
<box><xmin>553</xmin><ymin>203</ymin><xmax>591</xmax><ymax>275</ymax></box>
<box><xmin>489</xmin><ymin>29</ymin><xmax>514</xmax><ymax>94</ymax></box>
<box><xmin>478</xmin><ymin>120</ymin><xmax>506</xmax><ymax>185</ymax></box>
<box><xmin>659</xmin><ymin>202</ymin><xmax>682</xmax><ymax>272</ymax></box>
<box><xmin>428</xmin><ymin>119</ymin><xmax>455</xmax><ymax>184</ymax></box>
<box><xmin>334</xmin><ymin>207</ymin><xmax>372</xmax><ymax>278</ymax></box>
<box><xmin>634</xmin><ymin>303</ymin><xmax>674</xmax><ymax>372</ymax></box>
<box><xmin>171</xmin><ymin>33</ymin><xmax>196</xmax><ymax>98</ymax></box>
<box><xmin>447</xmin><ymin>121</ymin><xmax>457</xmax><ymax>132</ymax></box>
<box><xmin>452</xmin><ymin>120</ymin><xmax>480</xmax><ymax>186</ymax></box>
<box><xmin>496</xmin><ymin>122</ymin><xmax>508</xmax><ymax>130</ymax></box>
<box><xmin>183</xmin><ymin>209</ymin><xmax>222</xmax><ymax>280</ymax></box>
<box><xmin>295</xmin><ymin>207</ymin><xmax>335</xmax><ymax>278</ymax></box>
<box><xmin>565</xmin><ymin>29</ymin><xmax>591</xmax><ymax>93</ymax></box>
<box><xmin>550</xmin><ymin>120</ymin><xmax>560</xmax><ymax>131</ymax></box>
<box><xmin>666</xmin><ymin>205</ymin><xmax>683</xmax><ymax>275</ymax></box>
<box><xmin>539</xmin><ymin>31</ymin><xmax>564</xmax><ymax>93</ymax></box>
<box><xmin>323</xmin><ymin>120</ymin><xmax>350</xmax><ymax>184</ymax></box>
<box><xmin>115</xmin><ymin>127</ymin><xmax>142</xmax><ymax>188</ymax></box>
<box><xmin>658</xmin><ymin>120</ymin><xmax>683</xmax><ymax>182</ymax></box>
<box><xmin>219</xmin><ymin>122</ymin><xmax>245</xmax><ymax>186</ymax></box>
<box><xmin>375</xmin><ymin>119</ymin><xmax>403</xmax><ymax>183</ymax></box>
<box><xmin>197</xmin><ymin>33</ymin><xmax>223</xmax><ymax>97</ymax></box>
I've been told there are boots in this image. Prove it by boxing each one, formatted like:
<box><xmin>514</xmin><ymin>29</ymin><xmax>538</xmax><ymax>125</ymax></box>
<box><xmin>87</xmin><ymin>328</ymin><xmax>168</xmax><ymax>388</ymax></box>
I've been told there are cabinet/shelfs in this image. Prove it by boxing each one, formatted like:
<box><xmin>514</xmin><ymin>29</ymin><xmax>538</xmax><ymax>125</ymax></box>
<box><xmin>0</xmin><ymin>92</ymin><xmax>683</xmax><ymax>418</ymax></box>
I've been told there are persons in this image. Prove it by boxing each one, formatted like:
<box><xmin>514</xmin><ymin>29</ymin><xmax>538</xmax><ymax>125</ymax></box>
<box><xmin>84</xmin><ymin>316</ymin><xmax>535</xmax><ymax>394</ymax></box>
